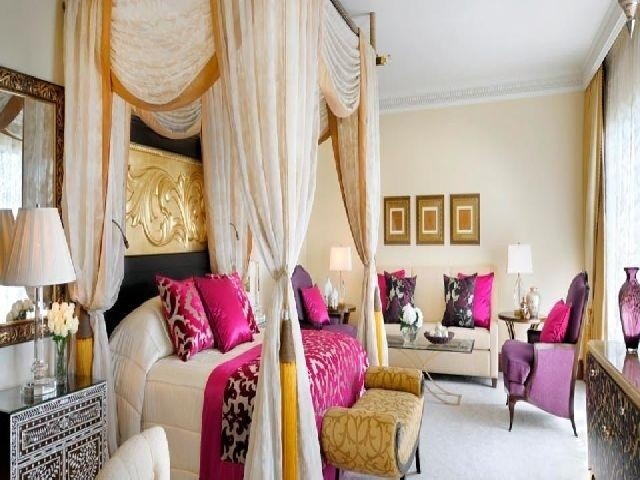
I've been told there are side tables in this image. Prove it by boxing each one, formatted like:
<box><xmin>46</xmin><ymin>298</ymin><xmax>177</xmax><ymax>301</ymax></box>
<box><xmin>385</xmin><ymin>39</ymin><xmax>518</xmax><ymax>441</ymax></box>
<box><xmin>327</xmin><ymin>305</ymin><xmax>356</xmax><ymax>325</ymax></box>
<box><xmin>498</xmin><ymin>311</ymin><xmax>548</xmax><ymax>341</ymax></box>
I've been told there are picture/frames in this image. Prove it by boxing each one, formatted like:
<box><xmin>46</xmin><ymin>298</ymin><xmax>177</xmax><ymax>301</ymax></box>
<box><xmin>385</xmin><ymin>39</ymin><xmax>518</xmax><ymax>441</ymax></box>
<box><xmin>450</xmin><ymin>194</ymin><xmax>480</xmax><ymax>246</ymax></box>
<box><xmin>416</xmin><ymin>194</ymin><xmax>445</xmax><ymax>246</ymax></box>
<box><xmin>383</xmin><ymin>195</ymin><xmax>411</xmax><ymax>245</ymax></box>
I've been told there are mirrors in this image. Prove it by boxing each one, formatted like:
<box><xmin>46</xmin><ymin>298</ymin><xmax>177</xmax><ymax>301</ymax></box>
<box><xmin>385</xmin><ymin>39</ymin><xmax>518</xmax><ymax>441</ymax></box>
<box><xmin>0</xmin><ymin>65</ymin><xmax>65</xmax><ymax>349</ymax></box>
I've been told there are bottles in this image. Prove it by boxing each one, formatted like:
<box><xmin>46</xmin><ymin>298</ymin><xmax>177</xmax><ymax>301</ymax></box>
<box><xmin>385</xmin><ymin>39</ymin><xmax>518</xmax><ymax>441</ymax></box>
<box><xmin>622</xmin><ymin>355</ymin><xmax>639</xmax><ymax>387</ymax></box>
<box><xmin>325</xmin><ymin>278</ymin><xmax>338</xmax><ymax>311</ymax></box>
<box><xmin>520</xmin><ymin>286</ymin><xmax>540</xmax><ymax>319</ymax></box>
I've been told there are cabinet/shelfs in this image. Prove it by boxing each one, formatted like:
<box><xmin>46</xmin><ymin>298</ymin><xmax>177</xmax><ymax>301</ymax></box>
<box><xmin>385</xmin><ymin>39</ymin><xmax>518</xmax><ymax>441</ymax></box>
<box><xmin>586</xmin><ymin>341</ymin><xmax>640</xmax><ymax>480</ymax></box>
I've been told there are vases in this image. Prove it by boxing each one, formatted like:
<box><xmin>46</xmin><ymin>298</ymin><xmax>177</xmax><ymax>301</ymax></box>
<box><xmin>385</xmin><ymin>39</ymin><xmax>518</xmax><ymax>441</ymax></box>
<box><xmin>54</xmin><ymin>334</ymin><xmax>69</xmax><ymax>386</ymax></box>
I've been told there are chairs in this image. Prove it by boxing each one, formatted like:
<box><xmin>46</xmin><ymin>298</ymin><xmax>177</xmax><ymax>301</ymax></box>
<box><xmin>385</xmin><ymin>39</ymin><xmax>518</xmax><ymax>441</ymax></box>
<box><xmin>291</xmin><ymin>265</ymin><xmax>358</xmax><ymax>339</ymax></box>
<box><xmin>501</xmin><ymin>271</ymin><xmax>590</xmax><ymax>438</ymax></box>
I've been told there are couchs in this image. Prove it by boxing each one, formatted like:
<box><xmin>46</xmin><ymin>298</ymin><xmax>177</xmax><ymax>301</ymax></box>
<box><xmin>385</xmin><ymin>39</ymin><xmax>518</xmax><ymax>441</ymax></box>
<box><xmin>377</xmin><ymin>265</ymin><xmax>500</xmax><ymax>388</ymax></box>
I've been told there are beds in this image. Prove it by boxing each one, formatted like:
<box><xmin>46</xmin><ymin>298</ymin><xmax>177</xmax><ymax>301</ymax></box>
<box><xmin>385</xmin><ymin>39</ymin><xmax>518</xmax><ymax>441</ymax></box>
<box><xmin>110</xmin><ymin>287</ymin><xmax>367</xmax><ymax>480</ymax></box>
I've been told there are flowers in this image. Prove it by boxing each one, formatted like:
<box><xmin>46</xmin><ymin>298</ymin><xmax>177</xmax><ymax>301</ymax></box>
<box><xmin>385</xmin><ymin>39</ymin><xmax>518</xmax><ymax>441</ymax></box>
<box><xmin>6</xmin><ymin>300</ymin><xmax>35</xmax><ymax>322</ymax></box>
<box><xmin>46</xmin><ymin>300</ymin><xmax>80</xmax><ymax>338</ymax></box>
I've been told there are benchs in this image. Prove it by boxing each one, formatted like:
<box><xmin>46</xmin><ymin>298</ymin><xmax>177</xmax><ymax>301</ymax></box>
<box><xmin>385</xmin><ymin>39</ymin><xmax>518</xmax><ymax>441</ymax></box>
<box><xmin>322</xmin><ymin>364</ymin><xmax>426</xmax><ymax>480</ymax></box>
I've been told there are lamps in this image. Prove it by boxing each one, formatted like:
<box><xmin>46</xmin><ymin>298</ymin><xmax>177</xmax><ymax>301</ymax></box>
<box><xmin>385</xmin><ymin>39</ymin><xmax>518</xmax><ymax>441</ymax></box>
<box><xmin>0</xmin><ymin>208</ymin><xmax>16</xmax><ymax>286</ymax></box>
<box><xmin>329</xmin><ymin>247</ymin><xmax>353</xmax><ymax>307</ymax></box>
<box><xmin>1</xmin><ymin>207</ymin><xmax>77</xmax><ymax>400</ymax></box>
<box><xmin>506</xmin><ymin>242</ymin><xmax>534</xmax><ymax>315</ymax></box>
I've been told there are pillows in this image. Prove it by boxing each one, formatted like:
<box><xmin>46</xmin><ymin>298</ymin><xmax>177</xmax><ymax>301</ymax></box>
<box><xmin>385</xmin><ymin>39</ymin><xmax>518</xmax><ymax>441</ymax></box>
<box><xmin>539</xmin><ymin>298</ymin><xmax>572</xmax><ymax>343</ymax></box>
<box><xmin>154</xmin><ymin>272</ymin><xmax>261</xmax><ymax>361</ymax></box>
<box><xmin>377</xmin><ymin>270</ymin><xmax>495</xmax><ymax>329</ymax></box>
<box><xmin>299</xmin><ymin>285</ymin><xmax>332</xmax><ymax>329</ymax></box>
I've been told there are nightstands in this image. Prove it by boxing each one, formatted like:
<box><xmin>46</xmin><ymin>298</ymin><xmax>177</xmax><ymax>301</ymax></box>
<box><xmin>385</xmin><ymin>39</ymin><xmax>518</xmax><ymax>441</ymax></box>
<box><xmin>0</xmin><ymin>373</ymin><xmax>109</xmax><ymax>480</ymax></box>
